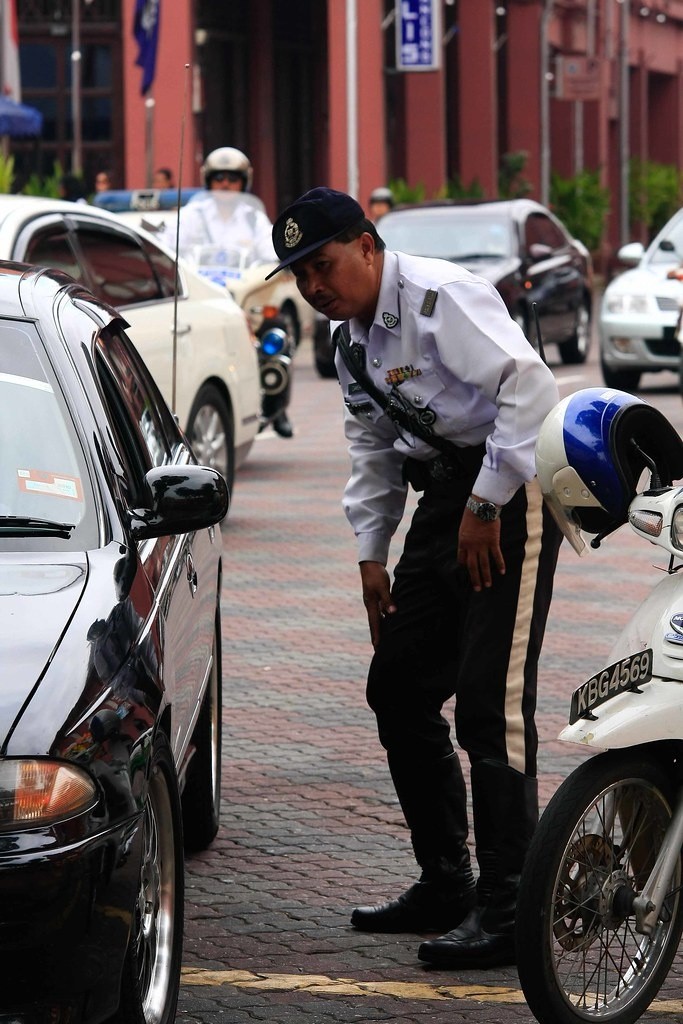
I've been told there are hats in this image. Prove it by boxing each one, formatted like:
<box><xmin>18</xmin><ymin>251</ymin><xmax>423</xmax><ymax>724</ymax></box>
<box><xmin>371</xmin><ymin>187</ymin><xmax>392</xmax><ymax>200</ymax></box>
<box><xmin>266</xmin><ymin>187</ymin><xmax>365</xmax><ymax>277</ymax></box>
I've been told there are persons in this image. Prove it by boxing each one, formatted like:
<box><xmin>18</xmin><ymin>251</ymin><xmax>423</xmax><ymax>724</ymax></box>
<box><xmin>264</xmin><ymin>184</ymin><xmax>561</xmax><ymax>972</ymax></box>
<box><xmin>365</xmin><ymin>186</ymin><xmax>396</xmax><ymax>222</ymax></box>
<box><xmin>94</xmin><ymin>170</ymin><xmax>111</xmax><ymax>192</ymax></box>
<box><xmin>199</xmin><ymin>146</ymin><xmax>294</xmax><ymax>440</ymax></box>
<box><xmin>151</xmin><ymin>168</ymin><xmax>173</xmax><ymax>189</ymax></box>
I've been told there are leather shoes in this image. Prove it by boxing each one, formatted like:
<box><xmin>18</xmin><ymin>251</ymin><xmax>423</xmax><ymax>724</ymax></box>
<box><xmin>351</xmin><ymin>881</ymin><xmax>519</xmax><ymax>969</ymax></box>
<box><xmin>262</xmin><ymin>408</ymin><xmax>292</xmax><ymax>437</ymax></box>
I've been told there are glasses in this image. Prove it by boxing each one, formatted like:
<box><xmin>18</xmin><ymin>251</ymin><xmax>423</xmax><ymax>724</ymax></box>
<box><xmin>211</xmin><ymin>172</ymin><xmax>239</xmax><ymax>182</ymax></box>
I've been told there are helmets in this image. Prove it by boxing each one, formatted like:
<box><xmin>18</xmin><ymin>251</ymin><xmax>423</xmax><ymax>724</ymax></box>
<box><xmin>199</xmin><ymin>147</ymin><xmax>253</xmax><ymax>192</ymax></box>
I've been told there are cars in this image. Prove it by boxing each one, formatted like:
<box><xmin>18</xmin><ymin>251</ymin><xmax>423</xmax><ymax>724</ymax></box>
<box><xmin>1</xmin><ymin>192</ymin><xmax>262</xmax><ymax>496</ymax></box>
<box><xmin>113</xmin><ymin>208</ymin><xmax>313</xmax><ymax>349</ymax></box>
<box><xmin>0</xmin><ymin>259</ymin><xmax>232</xmax><ymax>1024</ymax></box>
<box><xmin>309</xmin><ymin>198</ymin><xmax>594</xmax><ymax>378</ymax></box>
<box><xmin>599</xmin><ymin>206</ymin><xmax>683</xmax><ymax>394</ymax></box>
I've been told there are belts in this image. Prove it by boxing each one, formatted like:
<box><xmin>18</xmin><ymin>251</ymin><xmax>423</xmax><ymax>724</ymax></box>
<box><xmin>410</xmin><ymin>442</ymin><xmax>486</xmax><ymax>491</ymax></box>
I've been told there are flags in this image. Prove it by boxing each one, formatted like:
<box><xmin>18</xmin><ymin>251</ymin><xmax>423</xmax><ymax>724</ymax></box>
<box><xmin>3</xmin><ymin>0</ymin><xmax>21</xmax><ymax>104</ymax></box>
<box><xmin>132</xmin><ymin>0</ymin><xmax>160</xmax><ymax>95</ymax></box>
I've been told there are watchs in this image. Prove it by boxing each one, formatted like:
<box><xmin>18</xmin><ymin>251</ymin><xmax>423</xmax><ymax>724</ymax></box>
<box><xmin>465</xmin><ymin>496</ymin><xmax>502</xmax><ymax>522</ymax></box>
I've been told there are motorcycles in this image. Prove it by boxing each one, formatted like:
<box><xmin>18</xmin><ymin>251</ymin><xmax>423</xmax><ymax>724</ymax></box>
<box><xmin>512</xmin><ymin>432</ymin><xmax>683</xmax><ymax>1024</ymax></box>
<box><xmin>138</xmin><ymin>189</ymin><xmax>295</xmax><ymax>433</ymax></box>
